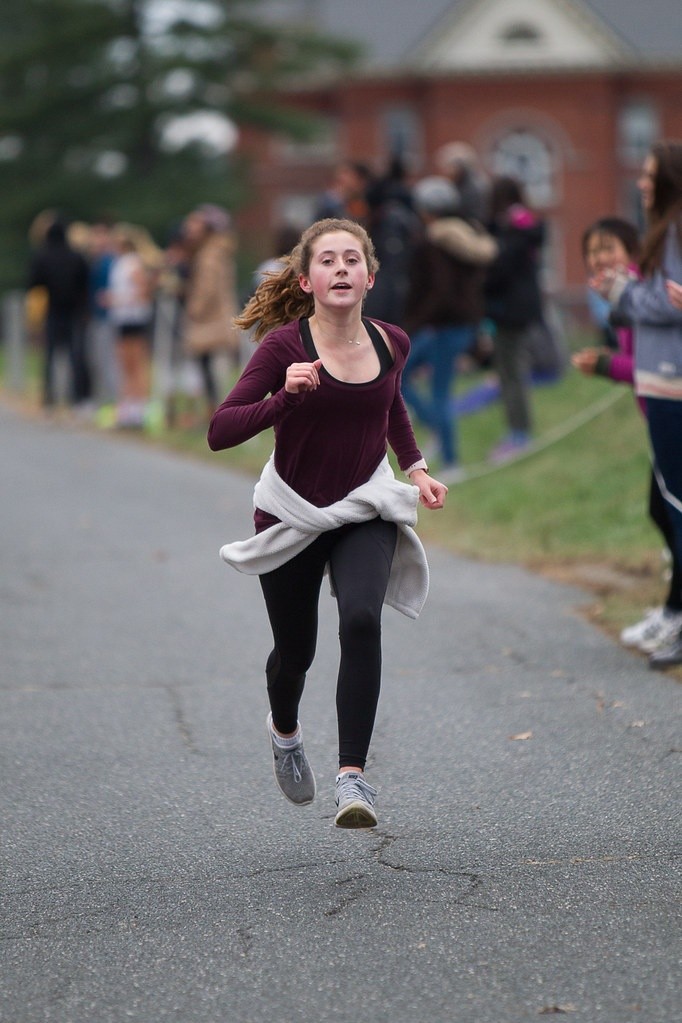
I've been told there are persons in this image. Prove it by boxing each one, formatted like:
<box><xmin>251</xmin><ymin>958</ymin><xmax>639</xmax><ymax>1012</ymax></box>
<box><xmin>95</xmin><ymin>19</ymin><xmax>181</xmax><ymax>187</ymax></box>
<box><xmin>21</xmin><ymin>142</ymin><xmax>565</xmax><ymax>470</ymax></box>
<box><xmin>207</xmin><ymin>217</ymin><xmax>448</xmax><ymax>829</ymax></box>
<box><xmin>591</xmin><ymin>141</ymin><xmax>681</xmax><ymax>666</ymax></box>
<box><xmin>570</xmin><ymin>212</ymin><xmax>649</xmax><ymax>416</ymax></box>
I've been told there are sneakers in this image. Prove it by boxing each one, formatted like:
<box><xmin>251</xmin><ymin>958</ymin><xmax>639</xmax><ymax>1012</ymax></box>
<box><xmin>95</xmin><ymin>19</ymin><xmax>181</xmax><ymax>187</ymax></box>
<box><xmin>332</xmin><ymin>771</ymin><xmax>379</xmax><ymax>829</ymax></box>
<box><xmin>648</xmin><ymin>627</ymin><xmax>682</xmax><ymax>670</ymax></box>
<box><xmin>266</xmin><ymin>711</ymin><xmax>316</xmax><ymax>806</ymax></box>
<box><xmin>621</xmin><ymin>607</ymin><xmax>680</xmax><ymax>654</ymax></box>
